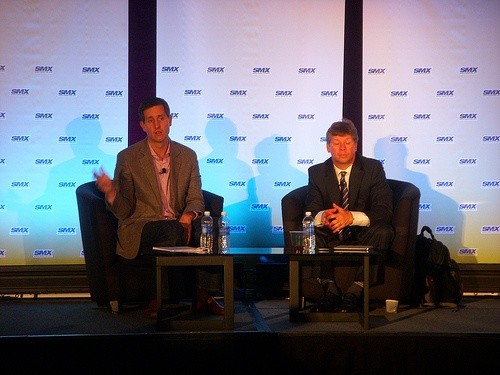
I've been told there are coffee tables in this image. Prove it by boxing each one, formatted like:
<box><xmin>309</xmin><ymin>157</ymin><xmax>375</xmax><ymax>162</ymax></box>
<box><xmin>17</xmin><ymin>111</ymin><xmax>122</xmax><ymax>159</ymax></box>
<box><xmin>281</xmin><ymin>244</ymin><xmax>378</xmax><ymax>331</ymax></box>
<box><xmin>154</xmin><ymin>246</ymin><xmax>237</xmax><ymax>327</ymax></box>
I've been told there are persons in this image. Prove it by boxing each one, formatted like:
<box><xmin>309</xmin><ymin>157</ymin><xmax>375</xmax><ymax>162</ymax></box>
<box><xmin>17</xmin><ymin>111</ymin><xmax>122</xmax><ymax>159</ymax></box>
<box><xmin>296</xmin><ymin>118</ymin><xmax>397</xmax><ymax>312</ymax></box>
<box><xmin>93</xmin><ymin>96</ymin><xmax>236</xmax><ymax>322</ymax></box>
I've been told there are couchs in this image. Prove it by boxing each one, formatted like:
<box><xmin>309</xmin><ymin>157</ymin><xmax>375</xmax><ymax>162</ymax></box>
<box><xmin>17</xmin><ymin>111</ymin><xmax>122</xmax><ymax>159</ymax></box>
<box><xmin>75</xmin><ymin>182</ymin><xmax>225</xmax><ymax>314</ymax></box>
<box><xmin>281</xmin><ymin>179</ymin><xmax>422</xmax><ymax>305</ymax></box>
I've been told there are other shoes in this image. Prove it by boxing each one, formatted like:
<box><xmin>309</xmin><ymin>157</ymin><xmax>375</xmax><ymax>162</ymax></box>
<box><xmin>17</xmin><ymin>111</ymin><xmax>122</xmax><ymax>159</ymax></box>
<box><xmin>310</xmin><ymin>292</ymin><xmax>342</xmax><ymax>312</ymax></box>
<box><xmin>144</xmin><ymin>299</ymin><xmax>158</xmax><ymax>318</ymax></box>
<box><xmin>192</xmin><ymin>293</ymin><xmax>224</xmax><ymax>315</ymax></box>
<box><xmin>338</xmin><ymin>293</ymin><xmax>364</xmax><ymax>311</ymax></box>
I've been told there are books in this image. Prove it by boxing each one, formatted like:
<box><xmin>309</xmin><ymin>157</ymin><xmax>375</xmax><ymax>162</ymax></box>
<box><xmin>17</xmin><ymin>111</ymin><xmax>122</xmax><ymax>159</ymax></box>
<box><xmin>151</xmin><ymin>245</ymin><xmax>200</xmax><ymax>253</ymax></box>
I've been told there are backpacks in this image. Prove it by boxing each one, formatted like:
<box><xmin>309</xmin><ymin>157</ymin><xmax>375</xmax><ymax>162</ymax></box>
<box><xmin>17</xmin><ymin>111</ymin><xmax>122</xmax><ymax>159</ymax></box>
<box><xmin>415</xmin><ymin>226</ymin><xmax>463</xmax><ymax>309</ymax></box>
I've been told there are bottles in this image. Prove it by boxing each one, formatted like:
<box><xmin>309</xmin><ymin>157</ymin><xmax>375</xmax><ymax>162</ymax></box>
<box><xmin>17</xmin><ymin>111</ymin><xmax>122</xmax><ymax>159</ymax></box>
<box><xmin>200</xmin><ymin>211</ymin><xmax>213</xmax><ymax>254</ymax></box>
<box><xmin>303</xmin><ymin>212</ymin><xmax>315</xmax><ymax>254</ymax></box>
<box><xmin>218</xmin><ymin>212</ymin><xmax>230</xmax><ymax>253</ymax></box>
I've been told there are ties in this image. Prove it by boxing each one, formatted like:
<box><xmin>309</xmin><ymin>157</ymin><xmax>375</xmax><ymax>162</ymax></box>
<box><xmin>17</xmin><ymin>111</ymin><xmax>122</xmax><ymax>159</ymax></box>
<box><xmin>338</xmin><ymin>171</ymin><xmax>352</xmax><ymax>242</ymax></box>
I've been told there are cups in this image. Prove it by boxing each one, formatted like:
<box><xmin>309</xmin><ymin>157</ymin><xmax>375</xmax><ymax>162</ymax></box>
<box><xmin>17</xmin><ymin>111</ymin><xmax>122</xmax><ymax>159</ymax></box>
<box><xmin>386</xmin><ymin>299</ymin><xmax>398</xmax><ymax>313</ymax></box>
<box><xmin>289</xmin><ymin>231</ymin><xmax>303</xmax><ymax>249</ymax></box>
